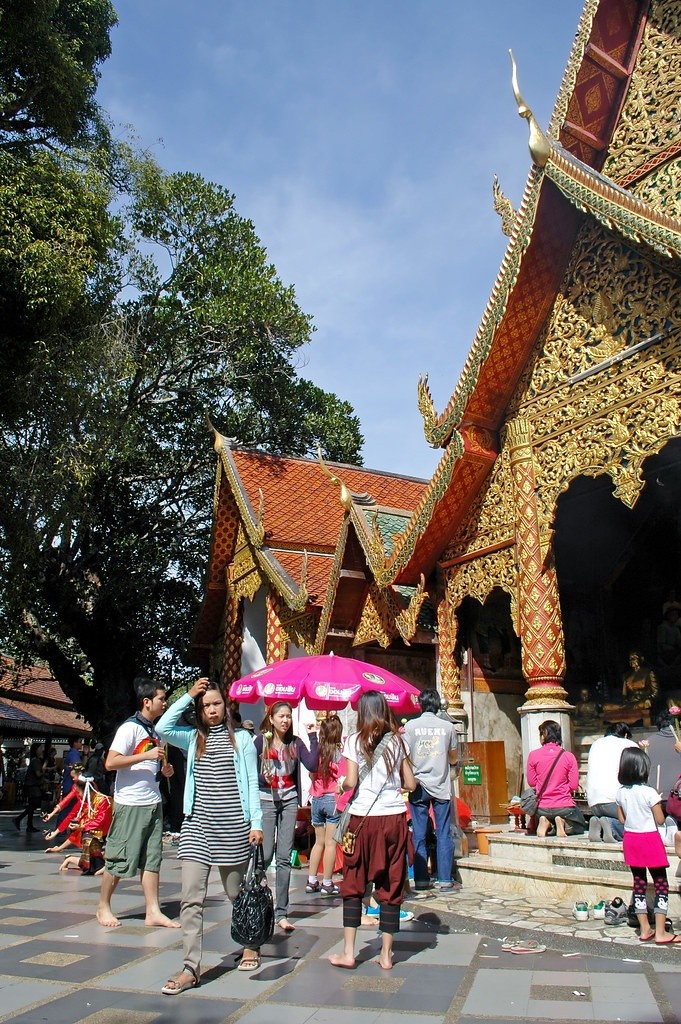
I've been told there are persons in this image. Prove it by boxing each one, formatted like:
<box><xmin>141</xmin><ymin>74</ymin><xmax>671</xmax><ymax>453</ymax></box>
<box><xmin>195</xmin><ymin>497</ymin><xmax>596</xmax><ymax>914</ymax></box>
<box><xmin>11</xmin><ymin>734</ymin><xmax>113</xmax><ymax>876</ymax></box>
<box><xmin>526</xmin><ymin>720</ymin><xmax>585</xmax><ymax>837</ymax></box>
<box><xmin>586</xmin><ymin>709</ymin><xmax>681</xmax><ymax>847</ymax></box>
<box><xmin>231</xmin><ymin>688</ymin><xmax>462</xmax><ymax>930</ymax></box>
<box><xmin>328</xmin><ymin>690</ymin><xmax>417</xmax><ymax>969</ymax></box>
<box><xmin>615</xmin><ymin>747</ymin><xmax>681</xmax><ymax>944</ymax></box>
<box><xmin>95</xmin><ymin>676</ymin><xmax>182</xmax><ymax>928</ymax></box>
<box><xmin>154</xmin><ymin>676</ymin><xmax>264</xmax><ymax>995</ymax></box>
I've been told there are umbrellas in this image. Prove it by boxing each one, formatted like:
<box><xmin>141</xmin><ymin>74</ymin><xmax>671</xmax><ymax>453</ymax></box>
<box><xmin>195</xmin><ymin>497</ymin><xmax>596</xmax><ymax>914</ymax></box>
<box><xmin>228</xmin><ymin>650</ymin><xmax>422</xmax><ymax>718</ymax></box>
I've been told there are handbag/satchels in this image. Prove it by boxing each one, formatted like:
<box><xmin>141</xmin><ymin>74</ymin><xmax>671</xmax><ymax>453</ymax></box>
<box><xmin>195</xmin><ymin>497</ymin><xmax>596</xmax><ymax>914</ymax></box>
<box><xmin>341</xmin><ymin>832</ymin><xmax>356</xmax><ymax>854</ymax></box>
<box><xmin>666</xmin><ymin>779</ymin><xmax>681</xmax><ymax>822</ymax></box>
<box><xmin>519</xmin><ymin>786</ymin><xmax>539</xmax><ymax>817</ymax></box>
<box><xmin>231</xmin><ymin>843</ymin><xmax>276</xmax><ymax>950</ymax></box>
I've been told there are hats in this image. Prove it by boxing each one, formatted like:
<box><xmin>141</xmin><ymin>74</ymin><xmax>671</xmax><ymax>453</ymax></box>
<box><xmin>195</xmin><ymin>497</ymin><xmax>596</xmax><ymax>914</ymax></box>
<box><xmin>95</xmin><ymin>743</ymin><xmax>104</xmax><ymax>749</ymax></box>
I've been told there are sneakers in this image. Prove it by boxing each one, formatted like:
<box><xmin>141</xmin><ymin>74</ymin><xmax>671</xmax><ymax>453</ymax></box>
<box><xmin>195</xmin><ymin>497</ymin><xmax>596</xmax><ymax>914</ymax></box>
<box><xmin>627</xmin><ymin>905</ymin><xmax>658</xmax><ymax>927</ymax></box>
<box><xmin>604</xmin><ymin>896</ymin><xmax>630</xmax><ymax>925</ymax></box>
<box><xmin>571</xmin><ymin>900</ymin><xmax>589</xmax><ymax>921</ymax></box>
<box><xmin>363</xmin><ymin>902</ymin><xmax>414</xmax><ymax>922</ymax></box>
<box><xmin>593</xmin><ymin>900</ymin><xmax>607</xmax><ymax>920</ymax></box>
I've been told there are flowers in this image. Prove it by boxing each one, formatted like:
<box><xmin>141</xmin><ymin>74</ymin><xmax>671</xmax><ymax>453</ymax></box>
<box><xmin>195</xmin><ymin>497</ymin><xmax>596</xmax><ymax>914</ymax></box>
<box><xmin>639</xmin><ymin>739</ymin><xmax>649</xmax><ymax>753</ymax></box>
<box><xmin>669</xmin><ymin>706</ymin><xmax>681</xmax><ymax>742</ymax></box>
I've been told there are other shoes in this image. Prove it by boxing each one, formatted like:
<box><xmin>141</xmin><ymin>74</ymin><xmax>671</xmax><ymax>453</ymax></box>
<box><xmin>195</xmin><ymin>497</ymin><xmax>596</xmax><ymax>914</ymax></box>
<box><xmin>439</xmin><ymin>880</ymin><xmax>463</xmax><ymax>891</ymax></box>
<box><xmin>414</xmin><ymin>881</ymin><xmax>436</xmax><ymax>890</ymax></box>
<box><xmin>13</xmin><ymin>818</ymin><xmax>21</xmax><ymax>832</ymax></box>
<box><xmin>26</xmin><ymin>827</ymin><xmax>40</xmax><ymax>833</ymax></box>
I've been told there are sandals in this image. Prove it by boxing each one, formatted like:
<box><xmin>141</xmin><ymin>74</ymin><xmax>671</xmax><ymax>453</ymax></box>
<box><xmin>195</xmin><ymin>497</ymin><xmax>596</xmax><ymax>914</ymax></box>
<box><xmin>237</xmin><ymin>946</ymin><xmax>261</xmax><ymax>971</ymax></box>
<box><xmin>304</xmin><ymin>880</ymin><xmax>321</xmax><ymax>893</ymax></box>
<box><xmin>321</xmin><ymin>882</ymin><xmax>341</xmax><ymax>895</ymax></box>
<box><xmin>161</xmin><ymin>965</ymin><xmax>202</xmax><ymax>995</ymax></box>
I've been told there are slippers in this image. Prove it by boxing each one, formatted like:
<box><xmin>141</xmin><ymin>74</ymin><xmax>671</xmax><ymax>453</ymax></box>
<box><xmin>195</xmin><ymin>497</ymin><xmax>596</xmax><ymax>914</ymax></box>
<box><xmin>638</xmin><ymin>929</ymin><xmax>656</xmax><ymax>941</ymax></box>
<box><xmin>655</xmin><ymin>933</ymin><xmax>681</xmax><ymax>944</ymax></box>
<box><xmin>501</xmin><ymin>936</ymin><xmax>547</xmax><ymax>954</ymax></box>
